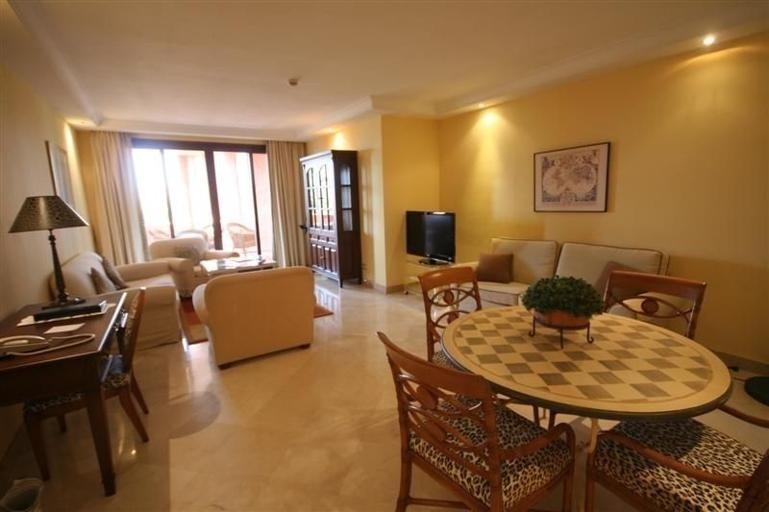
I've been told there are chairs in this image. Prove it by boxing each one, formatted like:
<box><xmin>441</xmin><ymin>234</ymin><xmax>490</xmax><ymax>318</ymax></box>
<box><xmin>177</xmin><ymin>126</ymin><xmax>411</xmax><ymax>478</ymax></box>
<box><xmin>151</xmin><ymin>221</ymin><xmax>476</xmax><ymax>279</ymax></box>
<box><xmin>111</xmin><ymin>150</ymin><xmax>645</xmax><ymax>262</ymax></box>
<box><xmin>22</xmin><ymin>287</ymin><xmax>149</xmax><ymax>480</ymax></box>
<box><xmin>376</xmin><ymin>330</ymin><xmax>576</xmax><ymax>511</ymax></box>
<box><xmin>227</xmin><ymin>222</ymin><xmax>256</xmax><ymax>254</ymax></box>
<box><xmin>148</xmin><ymin>227</ymin><xmax>169</xmax><ymax>240</ymax></box>
<box><xmin>417</xmin><ymin>267</ymin><xmax>542</xmax><ymax>428</ymax></box>
<box><xmin>583</xmin><ymin>379</ymin><xmax>769</xmax><ymax>512</ymax></box>
<box><xmin>202</xmin><ymin>223</ymin><xmax>224</xmax><ymax>250</ymax></box>
<box><xmin>602</xmin><ymin>269</ymin><xmax>708</xmax><ymax>342</ymax></box>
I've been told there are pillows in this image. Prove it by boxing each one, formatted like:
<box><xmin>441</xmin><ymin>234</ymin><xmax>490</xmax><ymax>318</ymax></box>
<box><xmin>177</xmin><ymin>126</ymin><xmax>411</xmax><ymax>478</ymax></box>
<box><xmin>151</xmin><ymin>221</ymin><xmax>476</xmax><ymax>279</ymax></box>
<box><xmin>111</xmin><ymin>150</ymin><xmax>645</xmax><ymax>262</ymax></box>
<box><xmin>475</xmin><ymin>250</ymin><xmax>514</xmax><ymax>284</ymax></box>
<box><xmin>594</xmin><ymin>259</ymin><xmax>649</xmax><ymax>311</ymax></box>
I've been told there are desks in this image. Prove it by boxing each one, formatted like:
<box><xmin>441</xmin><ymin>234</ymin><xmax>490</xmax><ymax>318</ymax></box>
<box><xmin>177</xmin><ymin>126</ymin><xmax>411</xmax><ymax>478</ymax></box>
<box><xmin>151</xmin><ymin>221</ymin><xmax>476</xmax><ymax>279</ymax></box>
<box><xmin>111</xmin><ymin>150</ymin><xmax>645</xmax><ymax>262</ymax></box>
<box><xmin>0</xmin><ymin>291</ymin><xmax>129</xmax><ymax>497</ymax></box>
<box><xmin>441</xmin><ymin>304</ymin><xmax>734</xmax><ymax>454</ymax></box>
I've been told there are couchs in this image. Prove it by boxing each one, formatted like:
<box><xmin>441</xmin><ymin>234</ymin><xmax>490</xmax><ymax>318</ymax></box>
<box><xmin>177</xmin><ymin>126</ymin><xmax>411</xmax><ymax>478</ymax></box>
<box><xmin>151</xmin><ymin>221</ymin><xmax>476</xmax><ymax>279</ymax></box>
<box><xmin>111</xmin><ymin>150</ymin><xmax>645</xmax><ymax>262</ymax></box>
<box><xmin>447</xmin><ymin>236</ymin><xmax>669</xmax><ymax>316</ymax></box>
<box><xmin>48</xmin><ymin>237</ymin><xmax>316</xmax><ymax>367</ymax></box>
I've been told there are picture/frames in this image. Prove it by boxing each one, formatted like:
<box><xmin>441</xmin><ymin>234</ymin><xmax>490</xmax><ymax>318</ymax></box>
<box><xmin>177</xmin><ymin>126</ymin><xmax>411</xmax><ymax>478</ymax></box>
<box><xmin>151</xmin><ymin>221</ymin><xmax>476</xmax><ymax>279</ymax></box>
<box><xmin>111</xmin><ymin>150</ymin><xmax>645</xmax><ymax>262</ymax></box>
<box><xmin>533</xmin><ymin>142</ymin><xmax>611</xmax><ymax>212</ymax></box>
<box><xmin>45</xmin><ymin>140</ymin><xmax>76</xmax><ymax>212</ymax></box>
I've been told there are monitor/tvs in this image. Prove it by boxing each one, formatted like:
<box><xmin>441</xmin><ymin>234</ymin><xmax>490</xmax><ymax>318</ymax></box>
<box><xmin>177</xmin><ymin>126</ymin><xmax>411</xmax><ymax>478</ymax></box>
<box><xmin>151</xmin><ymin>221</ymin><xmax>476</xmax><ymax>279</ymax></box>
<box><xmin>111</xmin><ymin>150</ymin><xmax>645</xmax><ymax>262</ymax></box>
<box><xmin>406</xmin><ymin>210</ymin><xmax>456</xmax><ymax>266</ymax></box>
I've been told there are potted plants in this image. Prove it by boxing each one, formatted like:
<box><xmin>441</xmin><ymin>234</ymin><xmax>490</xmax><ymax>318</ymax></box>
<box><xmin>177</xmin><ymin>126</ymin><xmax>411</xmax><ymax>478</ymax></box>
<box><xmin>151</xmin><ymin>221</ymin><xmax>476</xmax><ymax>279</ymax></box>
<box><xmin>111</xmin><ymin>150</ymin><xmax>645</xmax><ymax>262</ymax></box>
<box><xmin>520</xmin><ymin>274</ymin><xmax>605</xmax><ymax>349</ymax></box>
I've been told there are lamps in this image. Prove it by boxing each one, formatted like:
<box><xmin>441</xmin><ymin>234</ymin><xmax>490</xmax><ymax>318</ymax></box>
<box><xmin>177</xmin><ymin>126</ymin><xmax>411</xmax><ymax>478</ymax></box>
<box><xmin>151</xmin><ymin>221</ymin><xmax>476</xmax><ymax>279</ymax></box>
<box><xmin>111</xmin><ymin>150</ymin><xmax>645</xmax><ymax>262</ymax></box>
<box><xmin>9</xmin><ymin>194</ymin><xmax>92</xmax><ymax>311</ymax></box>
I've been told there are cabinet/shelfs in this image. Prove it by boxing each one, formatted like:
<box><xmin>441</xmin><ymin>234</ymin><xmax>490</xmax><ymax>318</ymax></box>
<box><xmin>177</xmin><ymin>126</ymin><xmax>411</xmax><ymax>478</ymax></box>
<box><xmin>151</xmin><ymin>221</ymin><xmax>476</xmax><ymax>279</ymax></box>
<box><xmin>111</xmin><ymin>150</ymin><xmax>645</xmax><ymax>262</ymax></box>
<box><xmin>404</xmin><ymin>260</ymin><xmax>455</xmax><ymax>303</ymax></box>
<box><xmin>299</xmin><ymin>149</ymin><xmax>364</xmax><ymax>288</ymax></box>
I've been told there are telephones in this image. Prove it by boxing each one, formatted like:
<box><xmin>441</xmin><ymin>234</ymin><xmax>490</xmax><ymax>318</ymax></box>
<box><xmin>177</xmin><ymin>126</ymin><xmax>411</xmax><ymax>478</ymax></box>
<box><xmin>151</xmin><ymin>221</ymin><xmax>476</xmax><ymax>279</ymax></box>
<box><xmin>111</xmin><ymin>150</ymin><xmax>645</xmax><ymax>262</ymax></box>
<box><xmin>0</xmin><ymin>333</ymin><xmax>54</xmax><ymax>352</ymax></box>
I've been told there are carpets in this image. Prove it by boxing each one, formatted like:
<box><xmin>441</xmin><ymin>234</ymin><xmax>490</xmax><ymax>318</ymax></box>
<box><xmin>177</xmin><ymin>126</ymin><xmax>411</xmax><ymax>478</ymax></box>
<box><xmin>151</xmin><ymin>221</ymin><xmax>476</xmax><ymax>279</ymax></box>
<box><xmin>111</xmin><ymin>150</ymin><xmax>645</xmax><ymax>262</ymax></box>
<box><xmin>178</xmin><ymin>296</ymin><xmax>334</xmax><ymax>345</ymax></box>
